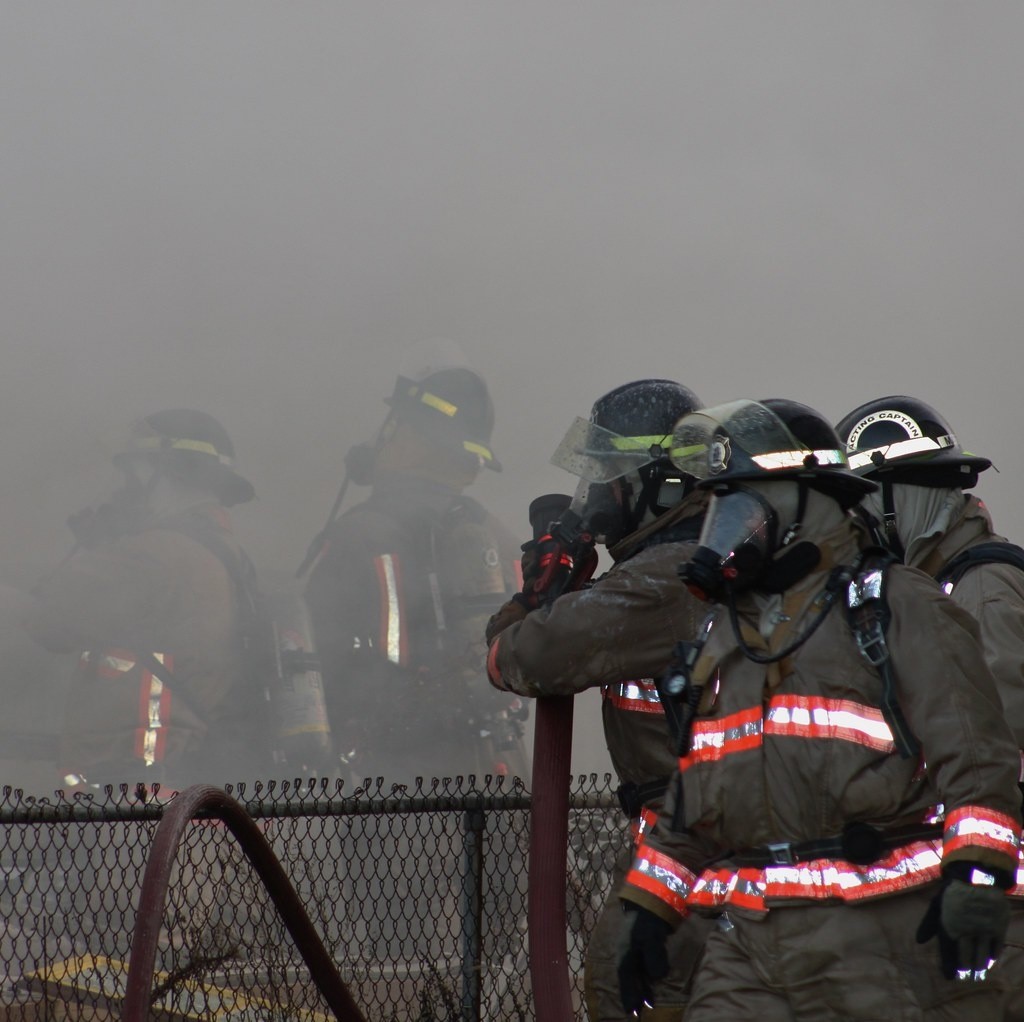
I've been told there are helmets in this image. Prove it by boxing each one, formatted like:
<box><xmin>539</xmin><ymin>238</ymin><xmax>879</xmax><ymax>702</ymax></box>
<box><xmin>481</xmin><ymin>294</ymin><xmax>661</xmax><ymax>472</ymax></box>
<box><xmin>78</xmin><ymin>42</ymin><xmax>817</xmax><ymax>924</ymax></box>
<box><xmin>550</xmin><ymin>378</ymin><xmax>708</xmax><ymax>485</ymax></box>
<box><xmin>669</xmin><ymin>397</ymin><xmax>877</xmax><ymax>509</ymax></box>
<box><xmin>405</xmin><ymin>369</ymin><xmax>506</xmax><ymax>474</ymax></box>
<box><xmin>835</xmin><ymin>394</ymin><xmax>991</xmax><ymax>489</ymax></box>
<box><xmin>112</xmin><ymin>408</ymin><xmax>255</xmax><ymax>507</ymax></box>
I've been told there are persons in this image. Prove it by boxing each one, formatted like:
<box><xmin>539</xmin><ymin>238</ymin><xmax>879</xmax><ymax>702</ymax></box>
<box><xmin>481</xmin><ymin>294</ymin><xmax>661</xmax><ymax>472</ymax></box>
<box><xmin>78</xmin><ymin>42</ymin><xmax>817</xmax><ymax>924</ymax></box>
<box><xmin>485</xmin><ymin>380</ymin><xmax>1023</xmax><ymax>1022</ymax></box>
<box><xmin>24</xmin><ymin>410</ymin><xmax>258</xmax><ymax>826</ymax></box>
<box><xmin>303</xmin><ymin>368</ymin><xmax>533</xmax><ymax>956</ymax></box>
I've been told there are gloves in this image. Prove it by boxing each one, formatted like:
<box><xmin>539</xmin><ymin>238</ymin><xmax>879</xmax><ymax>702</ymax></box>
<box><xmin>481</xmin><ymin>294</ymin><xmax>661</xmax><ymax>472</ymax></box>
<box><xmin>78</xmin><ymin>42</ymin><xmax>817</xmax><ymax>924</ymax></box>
<box><xmin>617</xmin><ymin>930</ymin><xmax>669</xmax><ymax>1018</ymax></box>
<box><xmin>915</xmin><ymin>880</ymin><xmax>1009</xmax><ymax>984</ymax></box>
<box><xmin>518</xmin><ymin>548</ymin><xmax>541</xmax><ymax>593</ymax></box>
